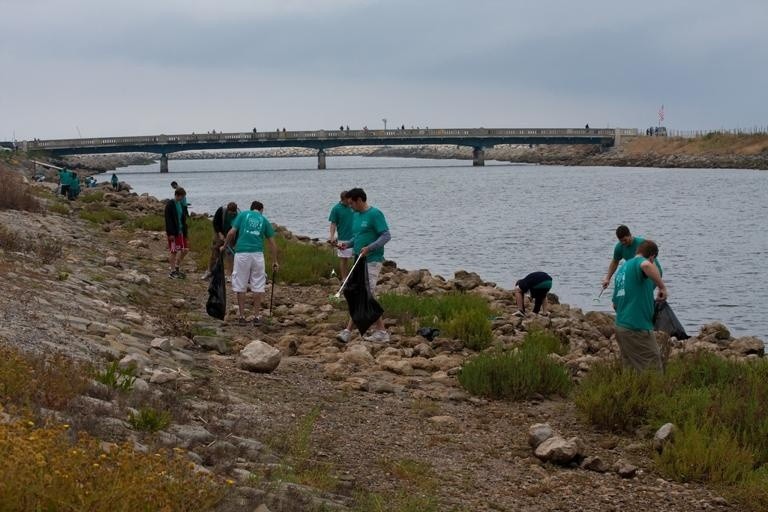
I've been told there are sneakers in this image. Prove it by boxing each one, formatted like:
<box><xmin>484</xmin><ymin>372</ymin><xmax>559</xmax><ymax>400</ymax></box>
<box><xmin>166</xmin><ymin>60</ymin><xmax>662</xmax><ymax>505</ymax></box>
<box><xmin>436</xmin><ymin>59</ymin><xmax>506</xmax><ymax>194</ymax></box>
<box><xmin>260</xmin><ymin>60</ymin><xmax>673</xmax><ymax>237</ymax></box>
<box><xmin>512</xmin><ymin>310</ymin><xmax>525</xmax><ymax>318</ymax></box>
<box><xmin>238</xmin><ymin>316</ymin><xmax>262</xmax><ymax>326</ymax></box>
<box><xmin>168</xmin><ymin>267</ymin><xmax>212</xmax><ymax>280</ymax></box>
<box><xmin>335</xmin><ymin>329</ymin><xmax>391</xmax><ymax>343</ymax></box>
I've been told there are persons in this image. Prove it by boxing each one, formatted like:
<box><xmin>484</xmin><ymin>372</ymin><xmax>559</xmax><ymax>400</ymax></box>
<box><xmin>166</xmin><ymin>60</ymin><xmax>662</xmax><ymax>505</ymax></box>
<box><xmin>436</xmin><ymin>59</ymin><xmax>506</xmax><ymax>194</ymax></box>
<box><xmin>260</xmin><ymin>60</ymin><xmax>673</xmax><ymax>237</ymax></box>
<box><xmin>585</xmin><ymin>122</ymin><xmax>590</xmax><ymax>133</ymax></box>
<box><xmin>601</xmin><ymin>225</ymin><xmax>662</xmax><ymax>290</ymax></box>
<box><xmin>57</xmin><ymin>166</ymin><xmax>94</xmax><ymax>200</ymax></box>
<box><xmin>219</xmin><ymin>201</ymin><xmax>279</xmax><ymax>326</ymax></box>
<box><xmin>171</xmin><ymin>181</ymin><xmax>188</xmax><ymax>235</ymax></box>
<box><xmin>511</xmin><ymin>272</ymin><xmax>552</xmax><ymax>317</ymax></box>
<box><xmin>335</xmin><ymin>188</ymin><xmax>391</xmax><ymax>344</ymax></box>
<box><xmin>328</xmin><ymin>191</ymin><xmax>354</xmax><ymax>284</ymax></box>
<box><xmin>200</xmin><ymin>202</ymin><xmax>242</xmax><ymax>282</ymax></box>
<box><xmin>165</xmin><ymin>187</ymin><xmax>190</xmax><ymax>280</ymax></box>
<box><xmin>111</xmin><ymin>173</ymin><xmax>120</xmax><ymax>192</ymax></box>
<box><xmin>192</xmin><ymin>125</ymin><xmax>429</xmax><ymax>142</ymax></box>
<box><xmin>612</xmin><ymin>241</ymin><xmax>667</xmax><ymax>375</ymax></box>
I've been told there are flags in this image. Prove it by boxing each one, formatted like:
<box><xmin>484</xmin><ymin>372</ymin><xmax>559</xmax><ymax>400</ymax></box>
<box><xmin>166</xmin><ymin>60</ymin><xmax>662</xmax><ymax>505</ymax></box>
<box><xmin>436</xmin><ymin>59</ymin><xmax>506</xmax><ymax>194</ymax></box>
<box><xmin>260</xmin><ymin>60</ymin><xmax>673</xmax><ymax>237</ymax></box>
<box><xmin>658</xmin><ymin>104</ymin><xmax>664</xmax><ymax>121</ymax></box>
